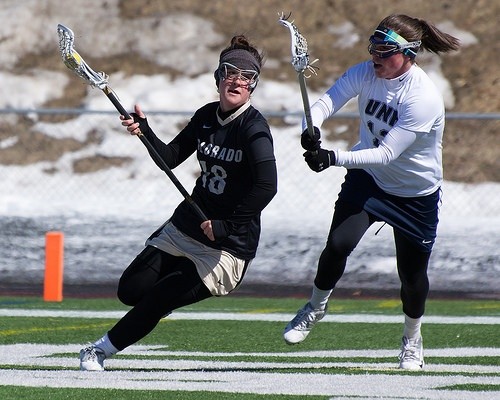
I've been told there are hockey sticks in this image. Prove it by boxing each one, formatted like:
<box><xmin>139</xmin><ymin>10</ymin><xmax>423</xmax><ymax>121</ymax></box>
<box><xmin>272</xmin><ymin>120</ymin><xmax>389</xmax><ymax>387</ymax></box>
<box><xmin>57</xmin><ymin>23</ymin><xmax>211</xmax><ymax>221</ymax></box>
<box><xmin>277</xmin><ymin>14</ymin><xmax>319</xmax><ymax>148</ymax></box>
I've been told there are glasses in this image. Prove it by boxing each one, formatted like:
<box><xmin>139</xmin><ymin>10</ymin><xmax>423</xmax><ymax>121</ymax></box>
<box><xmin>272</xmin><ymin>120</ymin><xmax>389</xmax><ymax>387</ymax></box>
<box><xmin>368</xmin><ymin>37</ymin><xmax>405</xmax><ymax>58</ymax></box>
<box><xmin>218</xmin><ymin>63</ymin><xmax>259</xmax><ymax>88</ymax></box>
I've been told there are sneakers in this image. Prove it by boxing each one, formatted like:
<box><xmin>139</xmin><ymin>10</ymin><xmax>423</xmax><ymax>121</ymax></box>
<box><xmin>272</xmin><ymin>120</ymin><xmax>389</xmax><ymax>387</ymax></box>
<box><xmin>282</xmin><ymin>302</ymin><xmax>329</xmax><ymax>345</ymax></box>
<box><xmin>80</xmin><ymin>346</ymin><xmax>107</xmax><ymax>373</ymax></box>
<box><xmin>398</xmin><ymin>336</ymin><xmax>425</xmax><ymax>370</ymax></box>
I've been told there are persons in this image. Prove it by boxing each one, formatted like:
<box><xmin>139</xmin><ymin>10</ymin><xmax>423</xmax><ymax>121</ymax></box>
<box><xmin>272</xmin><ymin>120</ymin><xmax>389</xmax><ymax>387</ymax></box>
<box><xmin>80</xmin><ymin>34</ymin><xmax>278</xmax><ymax>370</ymax></box>
<box><xmin>283</xmin><ymin>14</ymin><xmax>461</xmax><ymax>370</ymax></box>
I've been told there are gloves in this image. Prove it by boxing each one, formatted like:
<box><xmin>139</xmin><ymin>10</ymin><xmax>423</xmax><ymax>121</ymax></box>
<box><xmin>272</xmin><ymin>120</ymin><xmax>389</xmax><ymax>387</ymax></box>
<box><xmin>303</xmin><ymin>149</ymin><xmax>338</xmax><ymax>174</ymax></box>
<box><xmin>300</xmin><ymin>126</ymin><xmax>322</xmax><ymax>151</ymax></box>
